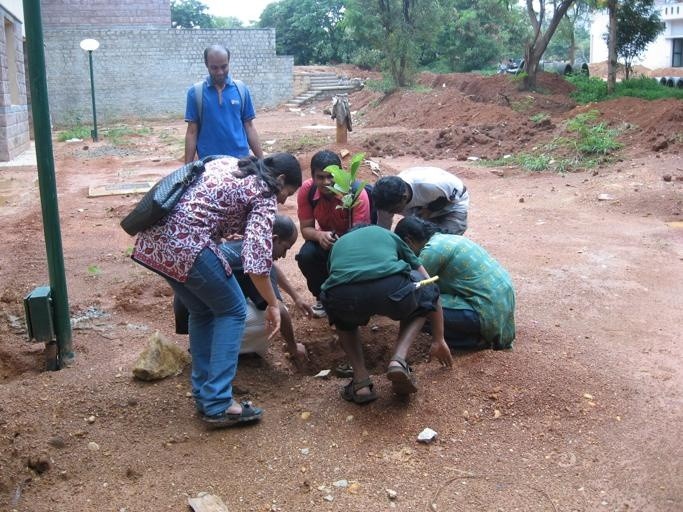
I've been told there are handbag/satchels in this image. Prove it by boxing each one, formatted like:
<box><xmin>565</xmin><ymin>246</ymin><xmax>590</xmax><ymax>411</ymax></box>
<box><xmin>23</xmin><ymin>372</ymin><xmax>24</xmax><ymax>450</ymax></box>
<box><xmin>120</xmin><ymin>155</ymin><xmax>236</xmax><ymax>239</ymax></box>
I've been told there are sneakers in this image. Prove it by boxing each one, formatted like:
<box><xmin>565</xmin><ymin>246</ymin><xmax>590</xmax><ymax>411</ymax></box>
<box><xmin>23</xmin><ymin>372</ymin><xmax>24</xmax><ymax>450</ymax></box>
<box><xmin>310</xmin><ymin>301</ymin><xmax>328</xmax><ymax>318</ymax></box>
<box><xmin>242</xmin><ymin>353</ymin><xmax>263</xmax><ymax>367</ymax></box>
<box><xmin>231</xmin><ymin>381</ymin><xmax>249</xmax><ymax>395</ymax></box>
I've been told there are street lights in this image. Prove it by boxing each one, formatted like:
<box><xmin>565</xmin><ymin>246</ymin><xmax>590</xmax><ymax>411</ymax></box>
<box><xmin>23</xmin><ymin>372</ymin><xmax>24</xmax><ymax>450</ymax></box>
<box><xmin>79</xmin><ymin>36</ymin><xmax>99</xmax><ymax>145</ymax></box>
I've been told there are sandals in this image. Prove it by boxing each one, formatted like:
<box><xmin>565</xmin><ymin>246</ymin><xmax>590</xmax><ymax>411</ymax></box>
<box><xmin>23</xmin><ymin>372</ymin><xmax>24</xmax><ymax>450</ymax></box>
<box><xmin>196</xmin><ymin>400</ymin><xmax>262</xmax><ymax>424</ymax></box>
<box><xmin>387</xmin><ymin>355</ymin><xmax>417</xmax><ymax>394</ymax></box>
<box><xmin>340</xmin><ymin>378</ymin><xmax>379</xmax><ymax>405</ymax></box>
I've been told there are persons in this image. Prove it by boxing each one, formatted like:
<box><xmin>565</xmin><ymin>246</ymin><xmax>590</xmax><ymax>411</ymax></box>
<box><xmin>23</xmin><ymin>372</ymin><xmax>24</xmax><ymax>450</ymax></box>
<box><xmin>173</xmin><ymin>215</ymin><xmax>298</xmax><ymax>396</ymax></box>
<box><xmin>372</xmin><ymin>164</ymin><xmax>469</xmax><ymax>236</ymax></box>
<box><xmin>131</xmin><ymin>152</ymin><xmax>302</xmax><ymax>424</ymax></box>
<box><xmin>394</xmin><ymin>215</ymin><xmax>516</xmax><ymax>351</ymax></box>
<box><xmin>184</xmin><ymin>43</ymin><xmax>264</xmax><ymax>163</ymax></box>
<box><xmin>297</xmin><ymin>149</ymin><xmax>371</xmax><ymax>318</ymax></box>
<box><xmin>319</xmin><ymin>223</ymin><xmax>454</xmax><ymax>405</ymax></box>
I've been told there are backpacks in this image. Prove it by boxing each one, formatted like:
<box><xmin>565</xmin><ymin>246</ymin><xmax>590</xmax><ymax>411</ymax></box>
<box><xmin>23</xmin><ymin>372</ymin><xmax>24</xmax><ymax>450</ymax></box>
<box><xmin>309</xmin><ymin>179</ymin><xmax>377</xmax><ymax>225</ymax></box>
